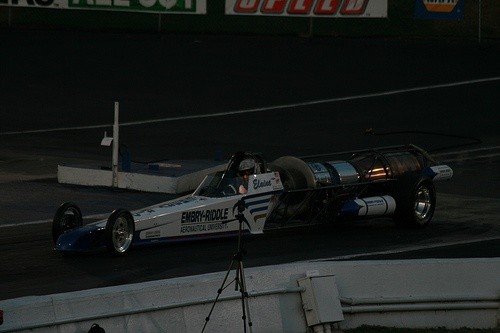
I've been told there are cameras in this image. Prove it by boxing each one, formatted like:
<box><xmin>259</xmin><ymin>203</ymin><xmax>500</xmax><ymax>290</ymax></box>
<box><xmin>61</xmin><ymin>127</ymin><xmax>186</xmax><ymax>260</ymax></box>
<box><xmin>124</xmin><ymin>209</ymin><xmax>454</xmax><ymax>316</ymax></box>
<box><xmin>235</xmin><ymin>200</ymin><xmax>246</xmax><ymax>222</ymax></box>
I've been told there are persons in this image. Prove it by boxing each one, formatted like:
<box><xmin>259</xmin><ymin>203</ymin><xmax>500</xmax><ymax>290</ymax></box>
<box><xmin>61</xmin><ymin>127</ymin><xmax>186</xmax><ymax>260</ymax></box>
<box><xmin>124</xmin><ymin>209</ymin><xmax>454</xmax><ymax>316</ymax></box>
<box><xmin>238</xmin><ymin>160</ymin><xmax>259</xmax><ymax>194</ymax></box>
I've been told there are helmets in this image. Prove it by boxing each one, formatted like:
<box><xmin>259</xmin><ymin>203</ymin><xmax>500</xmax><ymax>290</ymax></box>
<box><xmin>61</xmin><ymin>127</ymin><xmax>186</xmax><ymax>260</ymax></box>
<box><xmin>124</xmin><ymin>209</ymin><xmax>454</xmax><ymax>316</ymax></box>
<box><xmin>237</xmin><ymin>159</ymin><xmax>262</xmax><ymax>180</ymax></box>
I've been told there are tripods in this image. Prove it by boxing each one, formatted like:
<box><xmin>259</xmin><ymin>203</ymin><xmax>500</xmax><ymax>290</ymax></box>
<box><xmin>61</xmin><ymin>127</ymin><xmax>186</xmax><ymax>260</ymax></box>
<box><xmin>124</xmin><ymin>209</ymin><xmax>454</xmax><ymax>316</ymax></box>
<box><xmin>200</xmin><ymin>219</ymin><xmax>253</xmax><ymax>333</ymax></box>
